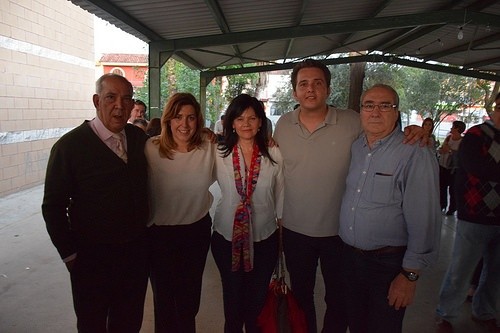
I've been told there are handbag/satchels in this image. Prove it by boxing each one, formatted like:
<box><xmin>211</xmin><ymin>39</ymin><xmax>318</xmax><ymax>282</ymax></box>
<box><xmin>255</xmin><ymin>222</ymin><xmax>309</xmax><ymax>333</ymax></box>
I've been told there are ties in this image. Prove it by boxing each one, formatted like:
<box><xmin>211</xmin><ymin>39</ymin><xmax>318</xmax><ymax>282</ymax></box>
<box><xmin>112</xmin><ymin>133</ymin><xmax>128</xmax><ymax>165</ymax></box>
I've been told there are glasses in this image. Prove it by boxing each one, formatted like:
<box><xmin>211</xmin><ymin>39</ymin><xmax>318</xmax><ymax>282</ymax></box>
<box><xmin>361</xmin><ymin>103</ymin><xmax>399</xmax><ymax>112</ymax></box>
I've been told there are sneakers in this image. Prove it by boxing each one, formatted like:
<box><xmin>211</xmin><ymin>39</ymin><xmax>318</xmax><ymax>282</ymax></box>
<box><xmin>435</xmin><ymin>319</ymin><xmax>454</xmax><ymax>333</ymax></box>
<box><xmin>470</xmin><ymin>312</ymin><xmax>500</xmax><ymax>333</ymax></box>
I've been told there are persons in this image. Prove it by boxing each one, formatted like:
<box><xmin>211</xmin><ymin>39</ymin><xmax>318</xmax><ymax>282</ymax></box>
<box><xmin>144</xmin><ymin>92</ymin><xmax>276</xmax><ymax>333</ymax></box>
<box><xmin>41</xmin><ymin>72</ymin><xmax>217</xmax><ymax>333</ymax></box>
<box><xmin>433</xmin><ymin>91</ymin><xmax>500</xmax><ymax>333</ymax></box>
<box><xmin>274</xmin><ymin>58</ymin><xmax>431</xmax><ymax>333</ymax></box>
<box><xmin>211</xmin><ymin>93</ymin><xmax>284</xmax><ymax>333</ymax></box>
<box><xmin>127</xmin><ymin>100</ymin><xmax>161</xmax><ymax>136</ymax></box>
<box><xmin>422</xmin><ymin>117</ymin><xmax>440</xmax><ymax>161</ymax></box>
<box><xmin>213</xmin><ymin>115</ymin><xmax>224</xmax><ymax>134</ymax></box>
<box><xmin>438</xmin><ymin>120</ymin><xmax>466</xmax><ymax>216</ymax></box>
<box><xmin>338</xmin><ymin>83</ymin><xmax>440</xmax><ymax>333</ymax></box>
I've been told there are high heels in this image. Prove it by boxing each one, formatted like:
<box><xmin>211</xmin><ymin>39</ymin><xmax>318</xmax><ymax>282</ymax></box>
<box><xmin>441</xmin><ymin>205</ymin><xmax>446</xmax><ymax>211</ymax></box>
<box><xmin>446</xmin><ymin>210</ymin><xmax>455</xmax><ymax>216</ymax></box>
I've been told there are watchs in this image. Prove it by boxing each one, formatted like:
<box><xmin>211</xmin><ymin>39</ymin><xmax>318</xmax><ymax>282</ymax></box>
<box><xmin>399</xmin><ymin>268</ymin><xmax>420</xmax><ymax>281</ymax></box>
